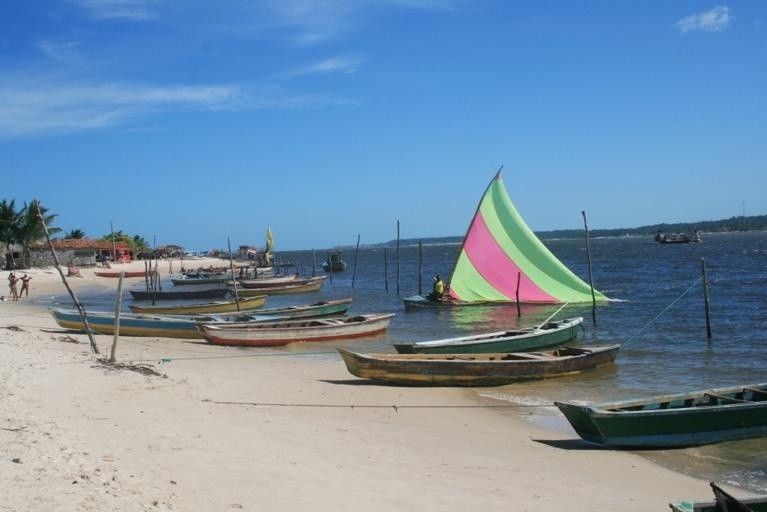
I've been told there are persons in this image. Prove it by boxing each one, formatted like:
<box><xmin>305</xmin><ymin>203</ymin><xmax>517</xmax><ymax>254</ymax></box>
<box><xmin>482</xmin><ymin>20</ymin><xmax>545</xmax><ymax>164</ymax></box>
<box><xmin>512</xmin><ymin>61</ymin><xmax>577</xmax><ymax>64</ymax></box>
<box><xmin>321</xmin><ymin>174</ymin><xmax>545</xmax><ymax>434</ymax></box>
<box><xmin>8</xmin><ymin>273</ymin><xmax>32</xmax><ymax>301</ymax></box>
<box><xmin>433</xmin><ymin>275</ymin><xmax>444</xmax><ymax>302</ymax></box>
<box><xmin>239</xmin><ymin>265</ymin><xmax>258</xmax><ymax>280</ymax></box>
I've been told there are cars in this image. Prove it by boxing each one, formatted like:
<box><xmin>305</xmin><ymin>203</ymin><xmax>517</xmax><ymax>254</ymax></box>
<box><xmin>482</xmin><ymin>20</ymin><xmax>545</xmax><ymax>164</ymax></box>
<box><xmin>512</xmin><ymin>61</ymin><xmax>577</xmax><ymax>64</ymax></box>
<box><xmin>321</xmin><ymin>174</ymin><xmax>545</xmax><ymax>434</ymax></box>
<box><xmin>96</xmin><ymin>251</ymin><xmax>168</xmax><ymax>262</ymax></box>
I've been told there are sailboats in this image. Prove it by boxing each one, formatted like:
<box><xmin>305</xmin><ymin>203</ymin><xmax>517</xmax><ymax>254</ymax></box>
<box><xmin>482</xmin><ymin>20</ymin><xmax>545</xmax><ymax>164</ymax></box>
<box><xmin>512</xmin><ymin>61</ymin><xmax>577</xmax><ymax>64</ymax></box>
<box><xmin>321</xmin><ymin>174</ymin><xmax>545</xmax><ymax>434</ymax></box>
<box><xmin>403</xmin><ymin>165</ymin><xmax>614</xmax><ymax>307</ymax></box>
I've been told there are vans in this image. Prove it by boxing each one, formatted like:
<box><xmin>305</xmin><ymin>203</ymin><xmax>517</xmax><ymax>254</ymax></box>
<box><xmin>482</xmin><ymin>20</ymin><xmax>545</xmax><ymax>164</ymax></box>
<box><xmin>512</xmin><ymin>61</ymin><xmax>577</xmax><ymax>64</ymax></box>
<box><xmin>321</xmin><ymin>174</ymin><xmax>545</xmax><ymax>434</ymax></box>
<box><xmin>2</xmin><ymin>251</ymin><xmax>20</xmax><ymax>269</ymax></box>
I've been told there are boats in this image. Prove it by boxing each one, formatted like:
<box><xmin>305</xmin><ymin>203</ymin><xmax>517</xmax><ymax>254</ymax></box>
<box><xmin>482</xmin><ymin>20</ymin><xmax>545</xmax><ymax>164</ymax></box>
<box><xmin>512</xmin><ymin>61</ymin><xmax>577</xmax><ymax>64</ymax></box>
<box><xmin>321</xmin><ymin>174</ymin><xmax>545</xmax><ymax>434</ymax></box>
<box><xmin>129</xmin><ymin>267</ymin><xmax>327</xmax><ymax>298</ymax></box>
<box><xmin>663</xmin><ymin>226</ymin><xmax>701</xmax><ymax>243</ymax></box>
<box><xmin>96</xmin><ymin>271</ymin><xmax>144</xmax><ymax>277</ymax></box>
<box><xmin>554</xmin><ymin>383</ymin><xmax>767</xmax><ymax>450</ymax></box>
<box><xmin>46</xmin><ymin>297</ymin><xmax>354</xmax><ymax>339</ymax></box>
<box><xmin>127</xmin><ymin>293</ymin><xmax>268</xmax><ymax>314</ymax></box>
<box><xmin>322</xmin><ymin>260</ymin><xmax>347</xmax><ymax>271</ymax></box>
<box><xmin>194</xmin><ymin>312</ymin><xmax>396</xmax><ymax>347</ymax></box>
<box><xmin>336</xmin><ymin>317</ymin><xmax>620</xmax><ymax>387</ymax></box>
